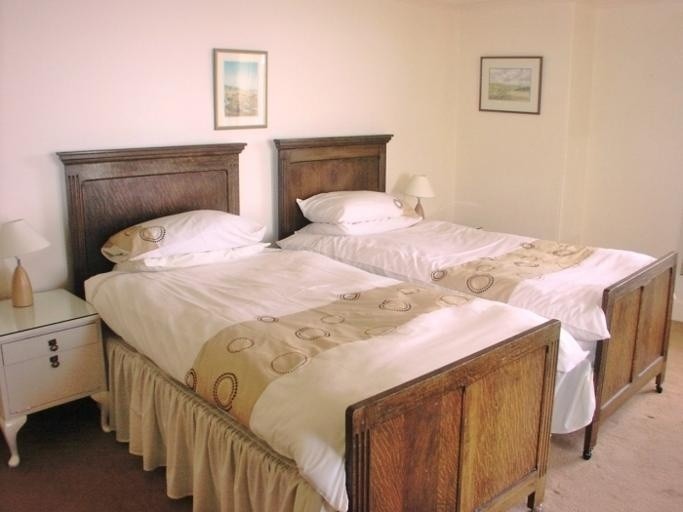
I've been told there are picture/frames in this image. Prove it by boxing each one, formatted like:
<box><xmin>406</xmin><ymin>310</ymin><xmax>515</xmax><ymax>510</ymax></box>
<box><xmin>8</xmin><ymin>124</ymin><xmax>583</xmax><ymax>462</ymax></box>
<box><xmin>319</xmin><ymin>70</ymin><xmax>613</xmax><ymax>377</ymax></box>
<box><xmin>212</xmin><ymin>48</ymin><xmax>269</xmax><ymax>131</ymax></box>
<box><xmin>479</xmin><ymin>56</ymin><xmax>543</xmax><ymax>115</ymax></box>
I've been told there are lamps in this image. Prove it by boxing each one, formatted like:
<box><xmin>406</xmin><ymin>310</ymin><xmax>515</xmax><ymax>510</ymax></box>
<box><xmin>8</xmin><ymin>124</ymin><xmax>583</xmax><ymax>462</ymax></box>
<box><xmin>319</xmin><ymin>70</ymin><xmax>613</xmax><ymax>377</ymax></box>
<box><xmin>405</xmin><ymin>175</ymin><xmax>434</xmax><ymax>221</ymax></box>
<box><xmin>1</xmin><ymin>220</ymin><xmax>51</xmax><ymax>308</ymax></box>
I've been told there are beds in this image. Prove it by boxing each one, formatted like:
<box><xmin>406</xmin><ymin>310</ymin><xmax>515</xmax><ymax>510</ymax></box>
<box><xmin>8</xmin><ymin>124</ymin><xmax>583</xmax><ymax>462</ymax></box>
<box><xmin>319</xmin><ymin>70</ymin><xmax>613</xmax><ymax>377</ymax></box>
<box><xmin>273</xmin><ymin>133</ymin><xmax>677</xmax><ymax>460</ymax></box>
<box><xmin>53</xmin><ymin>143</ymin><xmax>561</xmax><ymax>512</ymax></box>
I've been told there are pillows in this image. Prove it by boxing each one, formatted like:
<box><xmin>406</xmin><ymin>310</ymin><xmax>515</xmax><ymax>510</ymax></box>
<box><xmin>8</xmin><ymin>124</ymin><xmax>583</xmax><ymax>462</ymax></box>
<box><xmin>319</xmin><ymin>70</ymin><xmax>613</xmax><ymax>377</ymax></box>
<box><xmin>113</xmin><ymin>242</ymin><xmax>273</xmax><ymax>272</ymax></box>
<box><xmin>296</xmin><ymin>190</ymin><xmax>419</xmax><ymax>225</ymax></box>
<box><xmin>294</xmin><ymin>216</ymin><xmax>424</xmax><ymax>235</ymax></box>
<box><xmin>102</xmin><ymin>209</ymin><xmax>268</xmax><ymax>262</ymax></box>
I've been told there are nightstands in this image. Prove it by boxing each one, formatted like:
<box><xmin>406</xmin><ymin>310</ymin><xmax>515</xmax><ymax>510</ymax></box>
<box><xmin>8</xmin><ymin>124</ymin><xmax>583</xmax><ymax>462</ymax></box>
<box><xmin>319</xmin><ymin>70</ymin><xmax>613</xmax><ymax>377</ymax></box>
<box><xmin>0</xmin><ymin>286</ymin><xmax>113</xmax><ymax>467</ymax></box>
